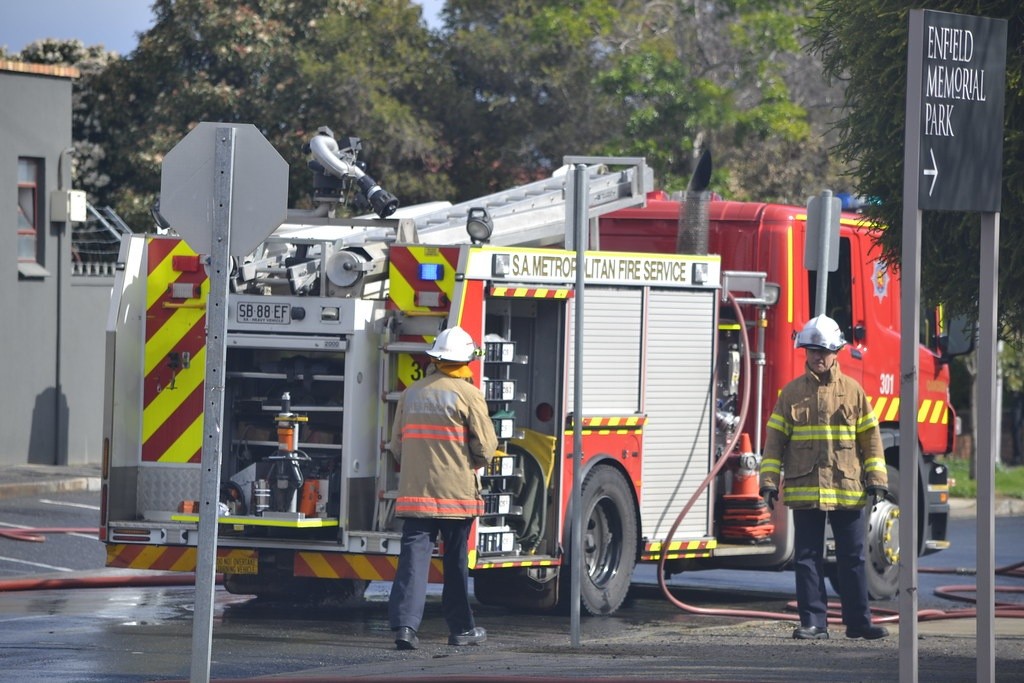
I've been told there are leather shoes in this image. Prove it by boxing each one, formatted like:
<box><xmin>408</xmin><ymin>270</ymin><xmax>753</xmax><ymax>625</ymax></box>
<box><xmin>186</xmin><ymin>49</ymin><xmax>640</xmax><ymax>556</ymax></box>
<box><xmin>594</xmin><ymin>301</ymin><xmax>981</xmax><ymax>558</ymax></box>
<box><xmin>449</xmin><ymin>625</ymin><xmax>487</xmax><ymax>646</ymax></box>
<box><xmin>792</xmin><ymin>621</ymin><xmax>830</xmax><ymax>641</ymax></box>
<box><xmin>846</xmin><ymin>623</ymin><xmax>888</xmax><ymax>639</ymax></box>
<box><xmin>395</xmin><ymin>627</ymin><xmax>420</xmax><ymax>651</ymax></box>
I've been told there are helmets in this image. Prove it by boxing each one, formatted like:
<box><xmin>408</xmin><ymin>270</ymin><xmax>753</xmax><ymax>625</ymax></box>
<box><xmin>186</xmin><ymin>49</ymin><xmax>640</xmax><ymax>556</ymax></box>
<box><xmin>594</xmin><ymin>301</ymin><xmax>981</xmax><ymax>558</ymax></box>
<box><xmin>798</xmin><ymin>314</ymin><xmax>847</xmax><ymax>350</ymax></box>
<box><xmin>425</xmin><ymin>325</ymin><xmax>475</xmax><ymax>363</ymax></box>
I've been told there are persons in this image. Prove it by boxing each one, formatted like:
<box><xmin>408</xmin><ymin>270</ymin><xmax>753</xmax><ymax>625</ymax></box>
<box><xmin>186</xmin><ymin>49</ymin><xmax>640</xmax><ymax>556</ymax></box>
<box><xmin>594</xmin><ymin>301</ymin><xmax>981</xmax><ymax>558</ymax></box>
<box><xmin>388</xmin><ymin>326</ymin><xmax>498</xmax><ymax>650</ymax></box>
<box><xmin>759</xmin><ymin>314</ymin><xmax>890</xmax><ymax>640</ymax></box>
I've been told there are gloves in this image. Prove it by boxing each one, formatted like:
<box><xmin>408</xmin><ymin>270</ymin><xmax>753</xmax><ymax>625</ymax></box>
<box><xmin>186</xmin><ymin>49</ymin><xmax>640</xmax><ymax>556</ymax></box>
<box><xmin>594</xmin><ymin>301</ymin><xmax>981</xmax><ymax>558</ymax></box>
<box><xmin>763</xmin><ymin>488</ymin><xmax>779</xmax><ymax>510</ymax></box>
<box><xmin>869</xmin><ymin>489</ymin><xmax>884</xmax><ymax>505</ymax></box>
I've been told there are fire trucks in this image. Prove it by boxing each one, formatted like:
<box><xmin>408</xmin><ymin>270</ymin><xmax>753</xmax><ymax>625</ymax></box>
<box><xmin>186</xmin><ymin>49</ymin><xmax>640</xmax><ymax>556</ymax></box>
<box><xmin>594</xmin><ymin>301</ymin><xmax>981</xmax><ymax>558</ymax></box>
<box><xmin>96</xmin><ymin>124</ymin><xmax>975</xmax><ymax>623</ymax></box>
